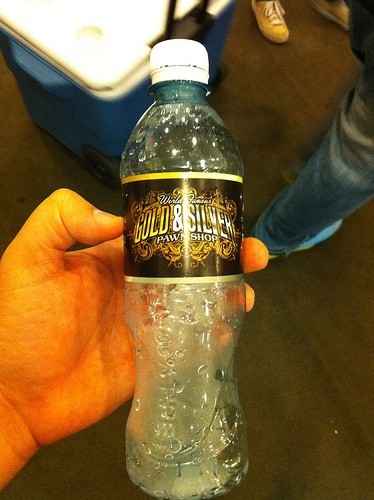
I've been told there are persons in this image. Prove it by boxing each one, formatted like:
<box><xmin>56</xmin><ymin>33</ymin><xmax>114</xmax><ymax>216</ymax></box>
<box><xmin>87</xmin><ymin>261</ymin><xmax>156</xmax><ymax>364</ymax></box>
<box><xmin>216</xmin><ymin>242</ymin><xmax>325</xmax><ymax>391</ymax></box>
<box><xmin>1</xmin><ymin>187</ymin><xmax>269</xmax><ymax>500</ymax></box>
<box><xmin>251</xmin><ymin>0</ymin><xmax>350</xmax><ymax>45</ymax></box>
<box><xmin>248</xmin><ymin>1</ymin><xmax>373</xmax><ymax>260</ymax></box>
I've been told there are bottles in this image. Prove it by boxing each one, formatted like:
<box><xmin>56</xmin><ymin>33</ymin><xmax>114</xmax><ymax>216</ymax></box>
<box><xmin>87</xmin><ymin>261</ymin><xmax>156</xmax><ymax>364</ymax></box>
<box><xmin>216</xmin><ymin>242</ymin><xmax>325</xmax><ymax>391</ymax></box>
<box><xmin>116</xmin><ymin>38</ymin><xmax>257</xmax><ymax>499</ymax></box>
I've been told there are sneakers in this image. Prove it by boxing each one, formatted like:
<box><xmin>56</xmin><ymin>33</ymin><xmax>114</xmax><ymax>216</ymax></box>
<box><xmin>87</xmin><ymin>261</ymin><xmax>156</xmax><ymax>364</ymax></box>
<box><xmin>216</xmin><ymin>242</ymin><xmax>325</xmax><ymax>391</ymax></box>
<box><xmin>252</xmin><ymin>0</ymin><xmax>290</xmax><ymax>44</ymax></box>
<box><xmin>310</xmin><ymin>0</ymin><xmax>351</xmax><ymax>32</ymax></box>
